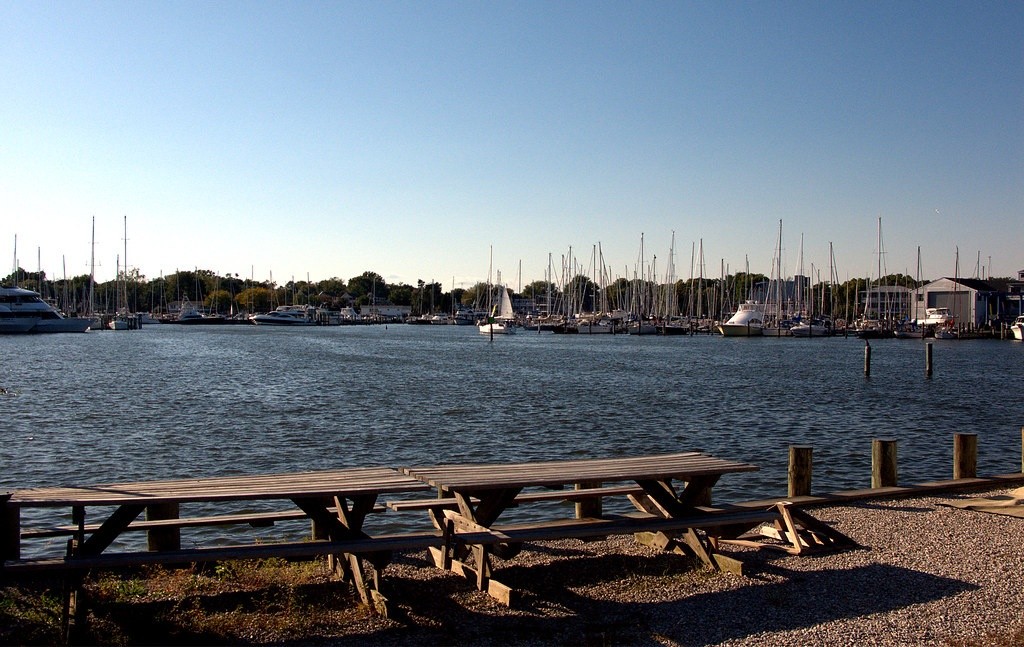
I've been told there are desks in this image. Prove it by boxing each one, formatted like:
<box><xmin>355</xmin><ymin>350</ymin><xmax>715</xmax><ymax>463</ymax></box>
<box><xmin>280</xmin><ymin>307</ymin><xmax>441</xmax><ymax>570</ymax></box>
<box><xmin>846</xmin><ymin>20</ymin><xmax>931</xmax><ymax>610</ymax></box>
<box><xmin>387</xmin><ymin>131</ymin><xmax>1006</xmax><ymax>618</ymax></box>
<box><xmin>399</xmin><ymin>454</ymin><xmax>761</xmax><ymax>605</ymax></box>
<box><xmin>7</xmin><ymin>466</ymin><xmax>431</xmax><ymax>647</ymax></box>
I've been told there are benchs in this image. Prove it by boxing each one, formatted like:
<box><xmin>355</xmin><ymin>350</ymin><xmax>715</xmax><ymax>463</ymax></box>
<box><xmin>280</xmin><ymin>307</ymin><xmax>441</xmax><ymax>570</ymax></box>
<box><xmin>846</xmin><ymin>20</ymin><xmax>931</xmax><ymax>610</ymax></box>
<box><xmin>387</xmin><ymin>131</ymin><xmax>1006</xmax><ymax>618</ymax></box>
<box><xmin>4</xmin><ymin>483</ymin><xmax>783</xmax><ymax>574</ymax></box>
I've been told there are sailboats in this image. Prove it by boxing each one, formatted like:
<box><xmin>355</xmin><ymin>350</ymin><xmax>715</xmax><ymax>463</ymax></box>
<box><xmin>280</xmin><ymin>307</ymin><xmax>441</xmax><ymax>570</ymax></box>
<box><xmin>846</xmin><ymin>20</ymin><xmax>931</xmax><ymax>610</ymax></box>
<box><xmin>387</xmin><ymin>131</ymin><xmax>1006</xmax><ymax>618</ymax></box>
<box><xmin>405</xmin><ymin>217</ymin><xmax>1024</xmax><ymax>340</ymax></box>
<box><xmin>13</xmin><ymin>215</ymin><xmax>401</xmax><ymax>325</ymax></box>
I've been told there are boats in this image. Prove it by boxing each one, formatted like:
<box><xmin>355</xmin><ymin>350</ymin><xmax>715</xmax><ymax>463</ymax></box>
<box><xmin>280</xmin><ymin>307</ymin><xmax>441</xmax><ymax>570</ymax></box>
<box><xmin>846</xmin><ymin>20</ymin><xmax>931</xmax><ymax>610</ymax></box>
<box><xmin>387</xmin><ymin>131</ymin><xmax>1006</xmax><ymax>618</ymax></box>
<box><xmin>0</xmin><ymin>282</ymin><xmax>99</xmax><ymax>335</ymax></box>
